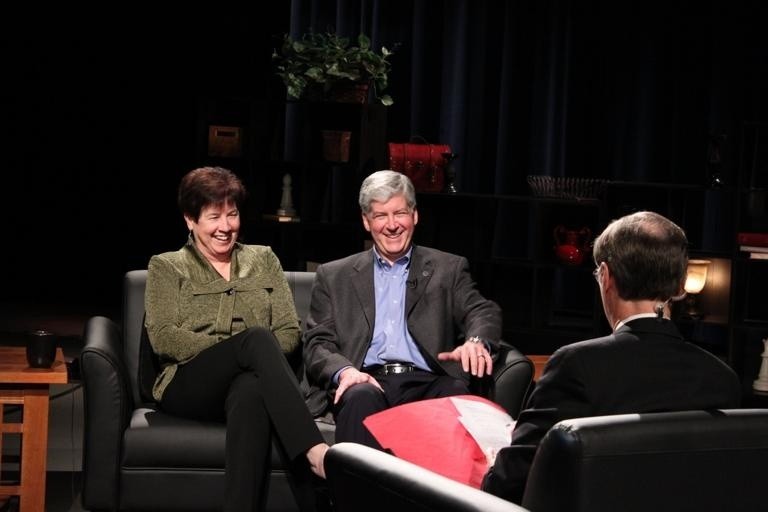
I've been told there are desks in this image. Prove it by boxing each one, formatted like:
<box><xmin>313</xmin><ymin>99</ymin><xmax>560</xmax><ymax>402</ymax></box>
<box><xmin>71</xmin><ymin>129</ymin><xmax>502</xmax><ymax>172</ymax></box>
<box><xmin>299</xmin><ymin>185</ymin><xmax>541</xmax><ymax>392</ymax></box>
<box><xmin>0</xmin><ymin>341</ymin><xmax>70</xmax><ymax>512</ymax></box>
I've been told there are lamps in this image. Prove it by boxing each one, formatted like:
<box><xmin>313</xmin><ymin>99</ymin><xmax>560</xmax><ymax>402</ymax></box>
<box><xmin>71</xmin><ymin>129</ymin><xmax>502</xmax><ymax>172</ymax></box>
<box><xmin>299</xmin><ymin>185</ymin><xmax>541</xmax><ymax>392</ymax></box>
<box><xmin>684</xmin><ymin>258</ymin><xmax>713</xmax><ymax>321</ymax></box>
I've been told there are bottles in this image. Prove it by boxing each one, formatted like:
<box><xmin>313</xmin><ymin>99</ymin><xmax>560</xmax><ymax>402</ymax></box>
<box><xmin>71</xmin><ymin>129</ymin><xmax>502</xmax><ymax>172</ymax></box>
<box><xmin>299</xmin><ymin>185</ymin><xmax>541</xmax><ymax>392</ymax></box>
<box><xmin>550</xmin><ymin>226</ymin><xmax>583</xmax><ymax>265</ymax></box>
<box><xmin>440</xmin><ymin>152</ymin><xmax>459</xmax><ymax>193</ymax></box>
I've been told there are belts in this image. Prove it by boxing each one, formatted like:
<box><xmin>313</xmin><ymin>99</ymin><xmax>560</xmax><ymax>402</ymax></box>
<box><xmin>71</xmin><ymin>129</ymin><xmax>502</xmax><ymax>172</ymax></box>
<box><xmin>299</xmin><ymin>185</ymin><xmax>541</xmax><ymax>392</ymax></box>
<box><xmin>367</xmin><ymin>362</ymin><xmax>428</xmax><ymax>376</ymax></box>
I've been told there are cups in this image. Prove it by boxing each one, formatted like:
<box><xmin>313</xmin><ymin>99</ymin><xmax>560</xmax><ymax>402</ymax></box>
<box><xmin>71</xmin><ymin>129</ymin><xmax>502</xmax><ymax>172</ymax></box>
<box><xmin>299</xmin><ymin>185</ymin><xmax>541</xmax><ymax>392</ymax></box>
<box><xmin>24</xmin><ymin>326</ymin><xmax>55</xmax><ymax>369</ymax></box>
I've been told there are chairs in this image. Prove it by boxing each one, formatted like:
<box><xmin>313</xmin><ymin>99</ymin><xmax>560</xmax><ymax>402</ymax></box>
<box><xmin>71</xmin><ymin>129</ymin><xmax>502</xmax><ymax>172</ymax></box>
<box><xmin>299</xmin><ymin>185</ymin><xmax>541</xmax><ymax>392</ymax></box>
<box><xmin>322</xmin><ymin>401</ymin><xmax>768</xmax><ymax>512</ymax></box>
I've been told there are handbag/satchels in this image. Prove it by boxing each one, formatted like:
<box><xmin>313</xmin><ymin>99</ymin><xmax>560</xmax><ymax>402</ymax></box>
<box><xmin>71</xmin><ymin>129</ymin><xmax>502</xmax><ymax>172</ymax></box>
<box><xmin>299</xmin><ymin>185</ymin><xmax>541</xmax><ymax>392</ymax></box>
<box><xmin>382</xmin><ymin>136</ymin><xmax>456</xmax><ymax>193</ymax></box>
<box><xmin>551</xmin><ymin>222</ymin><xmax>593</xmax><ymax>267</ymax></box>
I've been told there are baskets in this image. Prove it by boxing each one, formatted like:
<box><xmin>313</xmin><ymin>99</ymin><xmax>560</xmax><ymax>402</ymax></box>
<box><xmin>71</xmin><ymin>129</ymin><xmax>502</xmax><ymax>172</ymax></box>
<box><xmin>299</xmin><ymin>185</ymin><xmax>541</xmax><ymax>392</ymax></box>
<box><xmin>526</xmin><ymin>172</ymin><xmax>610</xmax><ymax>202</ymax></box>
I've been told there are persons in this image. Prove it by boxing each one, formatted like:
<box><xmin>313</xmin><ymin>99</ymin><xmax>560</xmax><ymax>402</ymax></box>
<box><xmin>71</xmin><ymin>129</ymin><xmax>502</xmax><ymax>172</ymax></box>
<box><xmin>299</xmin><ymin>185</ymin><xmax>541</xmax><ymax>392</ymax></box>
<box><xmin>481</xmin><ymin>210</ymin><xmax>741</xmax><ymax>504</ymax></box>
<box><xmin>142</xmin><ymin>166</ymin><xmax>330</xmax><ymax>512</ymax></box>
<box><xmin>301</xmin><ymin>167</ymin><xmax>505</xmax><ymax>451</ymax></box>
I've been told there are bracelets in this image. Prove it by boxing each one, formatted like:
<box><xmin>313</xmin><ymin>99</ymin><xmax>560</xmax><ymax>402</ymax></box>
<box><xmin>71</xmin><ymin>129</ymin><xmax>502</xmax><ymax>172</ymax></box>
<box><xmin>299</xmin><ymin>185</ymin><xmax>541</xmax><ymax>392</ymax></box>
<box><xmin>468</xmin><ymin>335</ymin><xmax>482</xmax><ymax>345</ymax></box>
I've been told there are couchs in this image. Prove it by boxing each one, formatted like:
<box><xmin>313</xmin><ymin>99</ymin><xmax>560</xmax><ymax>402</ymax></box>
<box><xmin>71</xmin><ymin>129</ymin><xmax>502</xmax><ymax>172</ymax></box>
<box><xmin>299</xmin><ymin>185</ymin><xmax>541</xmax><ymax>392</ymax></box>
<box><xmin>74</xmin><ymin>260</ymin><xmax>541</xmax><ymax>512</ymax></box>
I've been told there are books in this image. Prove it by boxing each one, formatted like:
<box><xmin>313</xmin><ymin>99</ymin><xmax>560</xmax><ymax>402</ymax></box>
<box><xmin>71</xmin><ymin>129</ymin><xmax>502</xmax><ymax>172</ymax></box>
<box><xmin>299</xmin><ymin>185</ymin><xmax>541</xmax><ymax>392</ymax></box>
<box><xmin>449</xmin><ymin>394</ymin><xmax>517</xmax><ymax>464</ymax></box>
